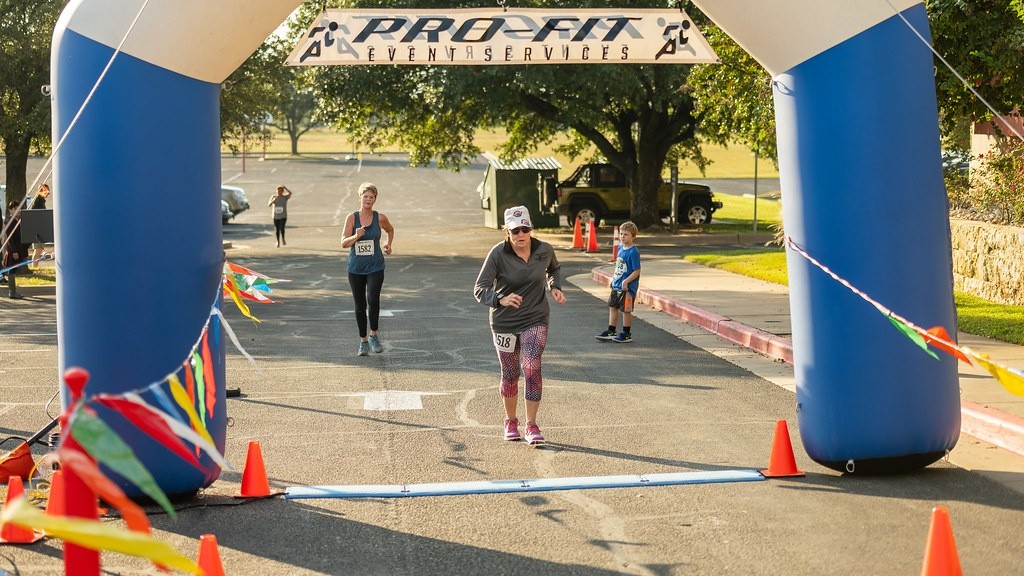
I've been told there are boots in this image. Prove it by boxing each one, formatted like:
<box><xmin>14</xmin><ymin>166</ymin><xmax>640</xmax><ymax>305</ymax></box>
<box><xmin>8</xmin><ymin>274</ymin><xmax>25</xmax><ymax>299</ymax></box>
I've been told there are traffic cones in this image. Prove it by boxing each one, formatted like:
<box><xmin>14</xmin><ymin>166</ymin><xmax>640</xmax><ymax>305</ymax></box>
<box><xmin>608</xmin><ymin>225</ymin><xmax>619</xmax><ymax>262</ymax></box>
<box><xmin>192</xmin><ymin>531</ymin><xmax>226</xmax><ymax>575</ymax></box>
<box><xmin>47</xmin><ymin>473</ymin><xmax>65</xmax><ymax>515</ymax></box>
<box><xmin>920</xmin><ymin>505</ymin><xmax>964</xmax><ymax>576</ymax></box>
<box><xmin>585</xmin><ymin>220</ymin><xmax>603</xmax><ymax>254</ymax></box>
<box><xmin>583</xmin><ymin>218</ymin><xmax>590</xmax><ymax>250</ymax></box>
<box><xmin>571</xmin><ymin>217</ymin><xmax>584</xmax><ymax>250</ymax></box>
<box><xmin>0</xmin><ymin>474</ymin><xmax>47</xmax><ymax>545</ymax></box>
<box><xmin>230</xmin><ymin>441</ymin><xmax>280</xmax><ymax>499</ymax></box>
<box><xmin>757</xmin><ymin>418</ymin><xmax>807</xmax><ymax>479</ymax></box>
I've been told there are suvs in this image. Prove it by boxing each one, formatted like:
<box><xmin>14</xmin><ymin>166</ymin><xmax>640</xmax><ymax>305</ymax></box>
<box><xmin>221</xmin><ymin>185</ymin><xmax>250</xmax><ymax>215</ymax></box>
<box><xmin>556</xmin><ymin>163</ymin><xmax>723</xmax><ymax>232</ymax></box>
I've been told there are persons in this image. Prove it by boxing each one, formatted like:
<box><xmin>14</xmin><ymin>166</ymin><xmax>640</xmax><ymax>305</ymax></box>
<box><xmin>341</xmin><ymin>183</ymin><xmax>394</xmax><ymax>357</ymax></box>
<box><xmin>31</xmin><ymin>184</ymin><xmax>50</xmax><ymax>267</ymax></box>
<box><xmin>3</xmin><ymin>200</ymin><xmax>29</xmax><ymax>299</ymax></box>
<box><xmin>473</xmin><ymin>206</ymin><xmax>564</xmax><ymax>445</ymax></box>
<box><xmin>267</xmin><ymin>186</ymin><xmax>292</xmax><ymax>248</ymax></box>
<box><xmin>0</xmin><ymin>207</ymin><xmax>8</xmax><ymax>284</ymax></box>
<box><xmin>593</xmin><ymin>222</ymin><xmax>641</xmax><ymax>343</ymax></box>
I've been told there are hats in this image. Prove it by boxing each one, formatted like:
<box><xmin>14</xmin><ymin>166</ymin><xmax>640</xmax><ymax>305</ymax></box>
<box><xmin>504</xmin><ymin>206</ymin><xmax>531</xmax><ymax>231</ymax></box>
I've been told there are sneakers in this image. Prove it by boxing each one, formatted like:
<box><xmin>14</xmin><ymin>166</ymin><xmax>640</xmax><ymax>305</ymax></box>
<box><xmin>368</xmin><ymin>334</ymin><xmax>383</xmax><ymax>353</ymax></box>
<box><xmin>612</xmin><ymin>332</ymin><xmax>632</xmax><ymax>342</ymax></box>
<box><xmin>595</xmin><ymin>329</ymin><xmax>618</xmax><ymax>340</ymax></box>
<box><xmin>358</xmin><ymin>340</ymin><xmax>370</xmax><ymax>356</ymax></box>
<box><xmin>525</xmin><ymin>425</ymin><xmax>545</xmax><ymax>446</ymax></box>
<box><xmin>503</xmin><ymin>418</ymin><xmax>521</xmax><ymax>441</ymax></box>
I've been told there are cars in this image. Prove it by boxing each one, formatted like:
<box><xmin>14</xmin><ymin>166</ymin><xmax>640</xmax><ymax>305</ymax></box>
<box><xmin>221</xmin><ymin>199</ymin><xmax>235</xmax><ymax>227</ymax></box>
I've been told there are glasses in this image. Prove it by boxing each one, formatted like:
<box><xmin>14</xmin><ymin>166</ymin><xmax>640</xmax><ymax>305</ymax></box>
<box><xmin>508</xmin><ymin>226</ymin><xmax>531</xmax><ymax>234</ymax></box>
